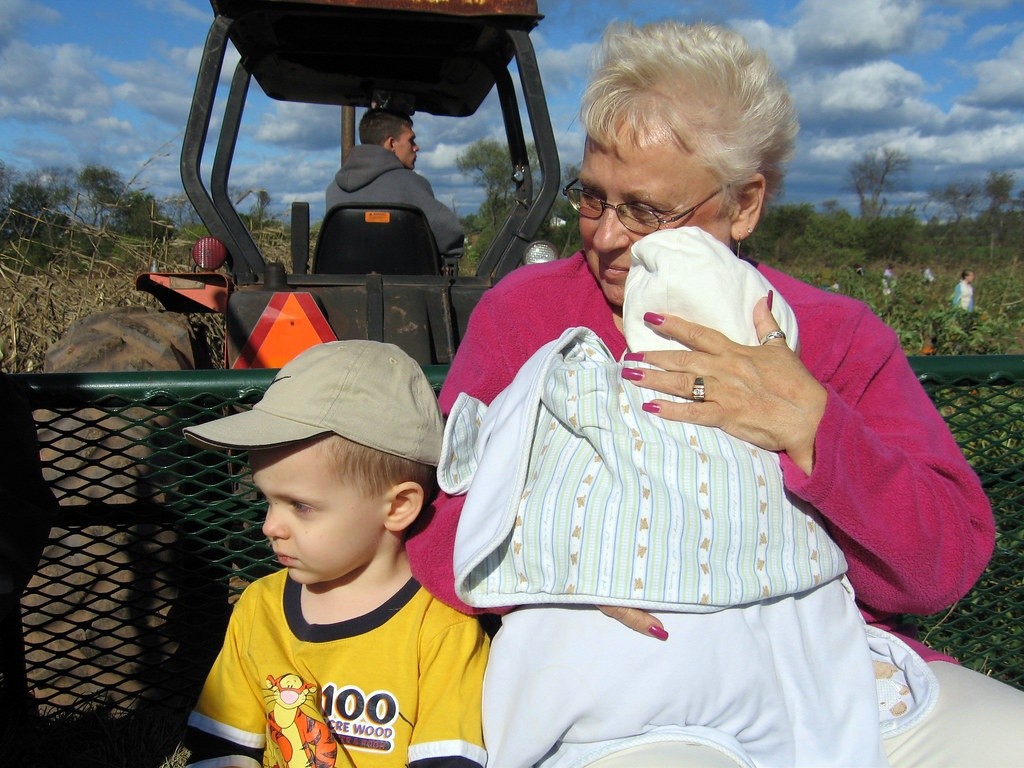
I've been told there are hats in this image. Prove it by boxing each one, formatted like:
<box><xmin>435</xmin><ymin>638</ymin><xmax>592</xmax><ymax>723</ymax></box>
<box><xmin>623</xmin><ymin>226</ymin><xmax>797</xmax><ymax>383</ymax></box>
<box><xmin>182</xmin><ymin>340</ymin><xmax>443</xmax><ymax>468</ymax></box>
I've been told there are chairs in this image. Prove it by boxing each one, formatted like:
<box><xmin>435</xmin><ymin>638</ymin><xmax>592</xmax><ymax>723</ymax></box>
<box><xmin>313</xmin><ymin>202</ymin><xmax>443</xmax><ymax>277</ymax></box>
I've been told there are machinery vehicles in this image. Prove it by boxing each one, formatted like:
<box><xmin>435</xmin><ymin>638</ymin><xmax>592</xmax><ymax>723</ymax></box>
<box><xmin>29</xmin><ymin>1</ymin><xmax>565</xmax><ymax>725</ymax></box>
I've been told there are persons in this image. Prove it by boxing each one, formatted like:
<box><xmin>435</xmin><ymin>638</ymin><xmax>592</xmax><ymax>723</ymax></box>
<box><xmin>325</xmin><ymin>107</ymin><xmax>467</xmax><ymax>272</ymax></box>
<box><xmin>179</xmin><ymin>340</ymin><xmax>494</xmax><ymax>768</ymax></box>
<box><xmin>405</xmin><ymin>18</ymin><xmax>1024</xmax><ymax>768</ymax></box>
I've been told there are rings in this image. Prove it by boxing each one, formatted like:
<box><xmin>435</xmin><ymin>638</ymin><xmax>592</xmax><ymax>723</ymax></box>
<box><xmin>691</xmin><ymin>374</ymin><xmax>706</xmax><ymax>403</ymax></box>
<box><xmin>758</xmin><ymin>329</ymin><xmax>786</xmax><ymax>346</ymax></box>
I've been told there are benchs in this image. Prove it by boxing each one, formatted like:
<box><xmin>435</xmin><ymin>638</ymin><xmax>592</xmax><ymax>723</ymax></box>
<box><xmin>0</xmin><ymin>353</ymin><xmax>1024</xmax><ymax>767</ymax></box>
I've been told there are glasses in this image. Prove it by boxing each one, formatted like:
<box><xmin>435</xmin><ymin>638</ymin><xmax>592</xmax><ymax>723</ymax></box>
<box><xmin>561</xmin><ymin>178</ymin><xmax>734</xmax><ymax>236</ymax></box>
